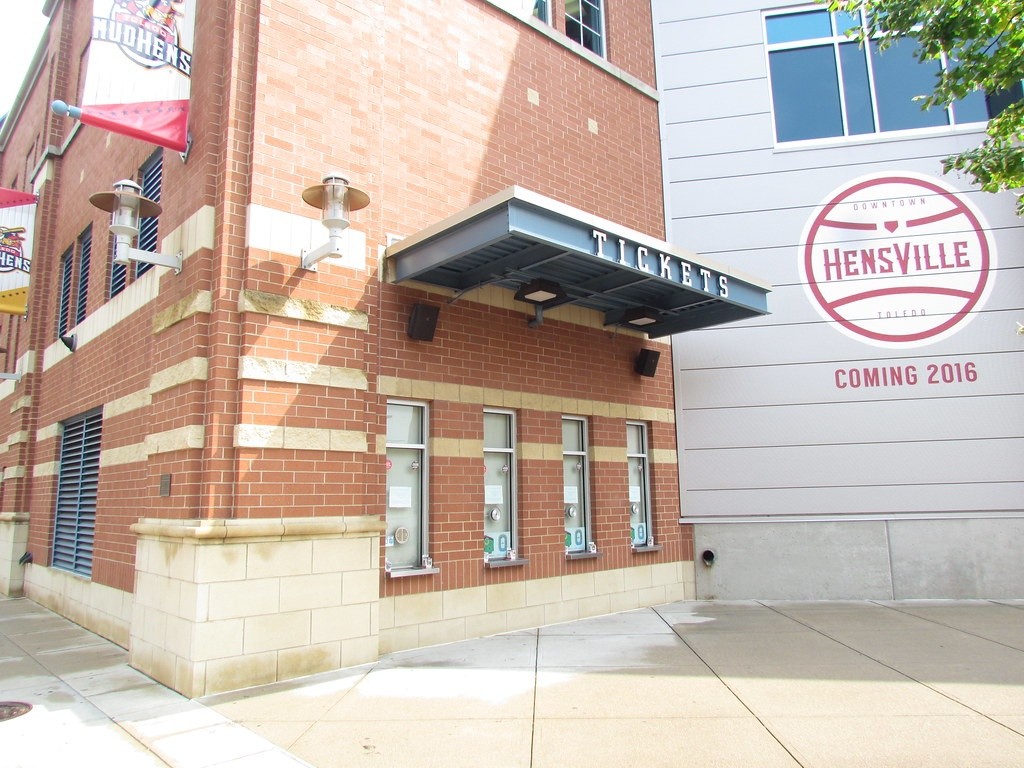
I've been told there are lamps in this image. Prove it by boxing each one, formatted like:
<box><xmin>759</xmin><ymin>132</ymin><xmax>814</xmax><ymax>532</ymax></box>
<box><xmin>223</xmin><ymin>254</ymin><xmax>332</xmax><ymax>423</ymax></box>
<box><xmin>88</xmin><ymin>179</ymin><xmax>182</xmax><ymax>275</ymax></box>
<box><xmin>301</xmin><ymin>173</ymin><xmax>370</xmax><ymax>270</ymax></box>
<box><xmin>514</xmin><ymin>279</ymin><xmax>565</xmax><ymax>304</ymax></box>
<box><xmin>619</xmin><ymin>306</ymin><xmax>665</xmax><ymax>328</ymax></box>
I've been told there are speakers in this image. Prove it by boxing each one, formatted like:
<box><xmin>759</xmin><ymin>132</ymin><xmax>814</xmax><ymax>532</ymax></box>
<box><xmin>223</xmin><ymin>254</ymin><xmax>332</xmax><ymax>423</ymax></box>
<box><xmin>408</xmin><ymin>303</ymin><xmax>439</xmax><ymax>342</ymax></box>
<box><xmin>635</xmin><ymin>348</ymin><xmax>660</xmax><ymax>377</ymax></box>
